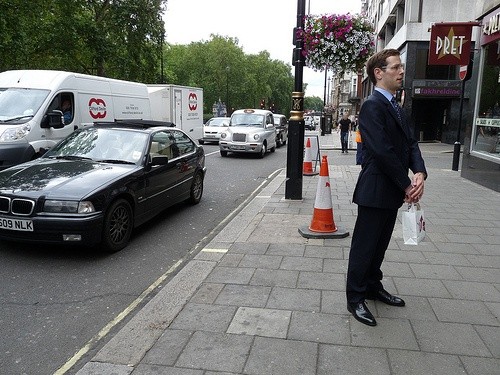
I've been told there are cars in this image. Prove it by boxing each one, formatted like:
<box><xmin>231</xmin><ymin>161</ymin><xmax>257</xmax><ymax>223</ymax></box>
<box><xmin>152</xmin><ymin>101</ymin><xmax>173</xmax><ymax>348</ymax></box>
<box><xmin>218</xmin><ymin>109</ymin><xmax>277</xmax><ymax>158</ymax></box>
<box><xmin>0</xmin><ymin>118</ymin><xmax>207</xmax><ymax>251</ymax></box>
<box><xmin>198</xmin><ymin>117</ymin><xmax>231</xmax><ymax>144</ymax></box>
<box><xmin>273</xmin><ymin>114</ymin><xmax>288</xmax><ymax>147</ymax></box>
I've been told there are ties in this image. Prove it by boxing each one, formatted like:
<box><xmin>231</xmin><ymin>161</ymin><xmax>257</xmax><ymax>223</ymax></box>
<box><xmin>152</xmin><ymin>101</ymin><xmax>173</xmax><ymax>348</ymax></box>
<box><xmin>391</xmin><ymin>97</ymin><xmax>402</xmax><ymax>122</ymax></box>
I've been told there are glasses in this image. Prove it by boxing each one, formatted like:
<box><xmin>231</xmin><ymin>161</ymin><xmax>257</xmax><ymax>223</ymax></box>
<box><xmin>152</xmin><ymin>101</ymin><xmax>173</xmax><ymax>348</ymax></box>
<box><xmin>380</xmin><ymin>64</ymin><xmax>404</xmax><ymax>72</ymax></box>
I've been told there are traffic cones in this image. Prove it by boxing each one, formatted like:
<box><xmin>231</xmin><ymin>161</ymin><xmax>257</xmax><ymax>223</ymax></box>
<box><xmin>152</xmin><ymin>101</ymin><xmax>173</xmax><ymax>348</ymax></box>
<box><xmin>303</xmin><ymin>138</ymin><xmax>319</xmax><ymax>176</ymax></box>
<box><xmin>298</xmin><ymin>156</ymin><xmax>349</xmax><ymax>239</ymax></box>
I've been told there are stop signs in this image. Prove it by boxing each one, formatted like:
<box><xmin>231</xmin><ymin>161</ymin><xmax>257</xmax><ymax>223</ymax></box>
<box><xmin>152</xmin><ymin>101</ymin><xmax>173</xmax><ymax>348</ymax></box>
<box><xmin>459</xmin><ymin>63</ymin><xmax>467</xmax><ymax>80</ymax></box>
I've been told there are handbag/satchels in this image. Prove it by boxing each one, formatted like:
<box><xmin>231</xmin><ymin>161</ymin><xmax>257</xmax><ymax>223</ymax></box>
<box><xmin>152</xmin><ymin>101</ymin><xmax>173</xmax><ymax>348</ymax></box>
<box><xmin>401</xmin><ymin>200</ymin><xmax>426</xmax><ymax>246</ymax></box>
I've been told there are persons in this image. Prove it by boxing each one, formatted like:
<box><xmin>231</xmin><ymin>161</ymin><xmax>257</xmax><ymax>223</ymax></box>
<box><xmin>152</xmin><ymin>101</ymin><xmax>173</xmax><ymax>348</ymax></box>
<box><xmin>355</xmin><ymin>122</ymin><xmax>364</xmax><ymax>166</ymax></box>
<box><xmin>337</xmin><ymin>114</ymin><xmax>351</xmax><ymax>153</ymax></box>
<box><xmin>344</xmin><ymin>48</ymin><xmax>427</xmax><ymax>326</ymax></box>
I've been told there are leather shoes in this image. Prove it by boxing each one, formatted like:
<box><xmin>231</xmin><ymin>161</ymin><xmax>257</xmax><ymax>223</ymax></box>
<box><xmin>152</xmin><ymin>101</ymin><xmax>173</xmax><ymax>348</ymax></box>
<box><xmin>369</xmin><ymin>284</ymin><xmax>405</xmax><ymax>307</ymax></box>
<box><xmin>347</xmin><ymin>301</ymin><xmax>376</xmax><ymax>326</ymax></box>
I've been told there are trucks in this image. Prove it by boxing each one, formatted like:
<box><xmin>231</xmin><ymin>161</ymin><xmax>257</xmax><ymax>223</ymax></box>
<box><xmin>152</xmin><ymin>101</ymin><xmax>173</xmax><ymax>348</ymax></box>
<box><xmin>0</xmin><ymin>70</ymin><xmax>204</xmax><ymax>172</ymax></box>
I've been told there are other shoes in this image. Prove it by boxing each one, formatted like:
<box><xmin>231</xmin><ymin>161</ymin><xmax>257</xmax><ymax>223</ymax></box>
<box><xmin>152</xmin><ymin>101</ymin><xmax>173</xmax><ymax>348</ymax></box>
<box><xmin>342</xmin><ymin>151</ymin><xmax>344</xmax><ymax>152</ymax></box>
<box><xmin>345</xmin><ymin>150</ymin><xmax>348</xmax><ymax>153</ymax></box>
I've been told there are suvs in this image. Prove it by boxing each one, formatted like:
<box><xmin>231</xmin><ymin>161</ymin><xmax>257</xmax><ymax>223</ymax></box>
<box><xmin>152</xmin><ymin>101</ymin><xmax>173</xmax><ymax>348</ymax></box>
<box><xmin>303</xmin><ymin>116</ymin><xmax>320</xmax><ymax>130</ymax></box>
